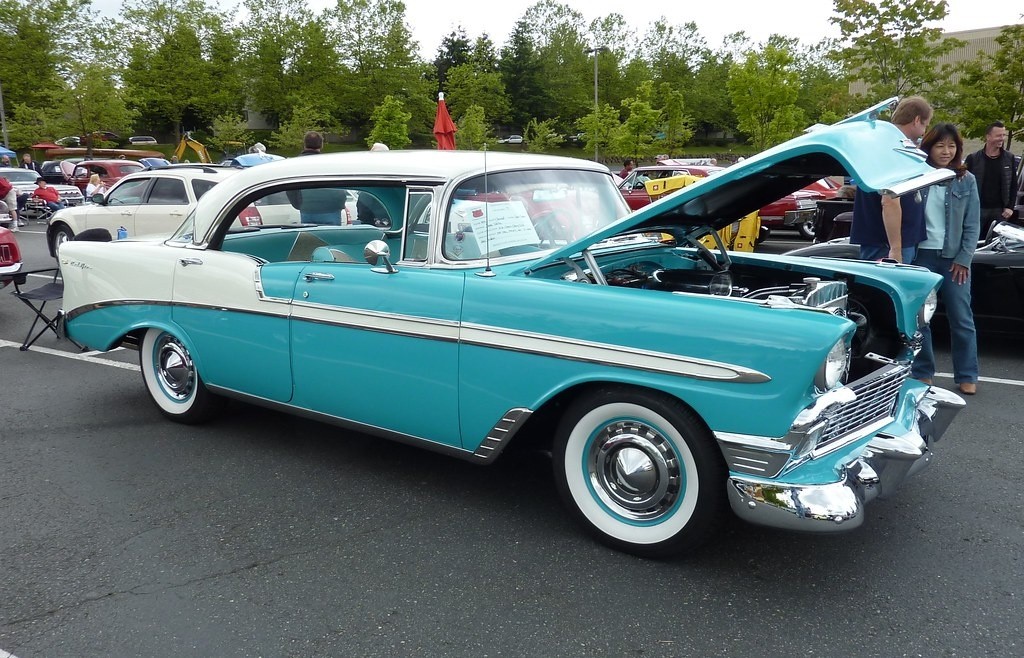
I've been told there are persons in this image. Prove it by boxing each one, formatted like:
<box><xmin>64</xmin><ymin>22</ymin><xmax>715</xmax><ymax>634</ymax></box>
<box><xmin>0</xmin><ymin>155</ymin><xmax>11</xmax><ymax>167</ymax></box>
<box><xmin>86</xmin><ymin>173</ymin><xmax>106</xmax><ymax>201</ymax></box>
<box><xmin>618</xmin><ymin>159</ymin><xmax>633</xmax><ymax>179</ymax></box>
<box><xmin>355</xmin><ymin>142</ymin><xmax>395</xmax><ymax>227</ymax></box>
<box><xmin>183</xmin><ymin>160</ymin><xmax>190</xmax><ymax>163</ymax></box>
<box><xmin>119</xmin><ymin>155</ymin><xmax>125</xmax><ymax>160</ymax></box>
<box><xmin>0</xmin><ymin>176</ymin><xmax>29</xmax><ymax>232</ymax></box>
<box><xmin>911</xmin><ymin>122</ymin><xmax>980</xmax><ymax>396</ymax></box>
<box><xmin>18</xmin><ymin>154</ymin><xmax>43</xmax><ymax>177</ymax></box>
<box><xmin>654</xmin><ymin>154</ymin><xmax>669</xmax><ymax>164</ymax></box>
<box><xmin>31</xmin><ymin>177</ymin><xmax>65</xmax><ymax>211</ymax></box>
<box><xmin>286</xmin><ymin>132</ymin><xmax>347</xmax><ymax>226</ymax></box>
<box><xmin>171</xmin><ymin>156</ymin><xmax>178</xmax><ymax>164</ymax></box>
<box><xmin>963</xmin><ymin>122</ymin><xmax>1017</xmax><ymax>238</ymax></box>
<box><xmin>849</xmin><ymin>95</ymin><xmax>931</xmax><ymax>266</ymax></box>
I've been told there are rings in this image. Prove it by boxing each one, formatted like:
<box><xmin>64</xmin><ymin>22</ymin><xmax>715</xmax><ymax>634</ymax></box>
<box><xmin>959</xmin><ymin>272</ymin><xmax>963</xmax><ymax>275</ymax></box>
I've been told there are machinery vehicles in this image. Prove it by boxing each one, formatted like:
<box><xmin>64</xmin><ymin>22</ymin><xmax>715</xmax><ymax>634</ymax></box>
<box><xmin>172</xmin><ymin>130</ymin><xmax>212</xmax><ymax>163</ymax></box>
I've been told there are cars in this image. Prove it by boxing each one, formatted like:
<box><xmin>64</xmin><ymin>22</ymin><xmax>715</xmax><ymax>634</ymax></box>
<box><xmin>55</xmin><ymin>136</ymin><xmax>80</xmax><ymax>147</ymax></box>
<box><xmin>67</xmin><ymin>158</ymin><xmax>148</xmax><ymax>203</ymax></box>
<box><xmin>0</xmin><ymin>167</ymin><xmax>84</xmax><ymax>218</ymax></box>
<box><xmin>138</xmin><ymin>158</ymin><xmax>171</xmax><ymax>167</ymax></box>
<box><xmin>784</xmin><ymin>219</ymin><xmax>1024</xmax><ymax>353</ymax></box>
<box><xmin>610</xmin><ymin>158</ymin><xmax>845</xmax><ymax>240</ymax></box>
<box><xmin>220</xmin><ymin>153</ymin><xmax>285</xmax><ymax>168</ymax></box>
<box><xmin>80</xmin><ymin>131</ymin><xmax>122</xmax><ymax>147</ymax></box>
<box><xmin>56</xmin><ymin>94</ymin><xmax>967</xmax><ymax>562</ymax></box>
<box><xmin>498</xmin><ymin>134</ymin><xmax>523</xmax><ymax>144</ymax></box>
<box><xmin>46</xmin><ymin>163</ymin><xmax>352</xmax><ymax>262</ymax></box>
<box><xmin>0</xmin><ymin>224</ymin><xmax>23</xmax><ymax>293</ymax></box>
<box><xmin>41</xmin><ymin>160</ymin><xmax>66</xmax><ymax>184</ymax></box>
<box><xmin>468</xmin><ymin>181</ymin><xmax>599</xmax><ymax>241</ymax></box>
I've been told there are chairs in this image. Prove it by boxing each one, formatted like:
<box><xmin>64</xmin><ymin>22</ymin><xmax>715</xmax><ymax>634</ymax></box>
<box><xmin>11</xmin><ymin>256</ymin><xmax>90</xmax><ymax>352</ymax></box>
<box><xmin>10</xmin><ymin>186</ymin><xmax>31</xmax><ymax>226</ymax></box>
<box><xmin>33</xmin><ymin>193</ymin><xmax>70</xmax><ymax>225</ymax></box>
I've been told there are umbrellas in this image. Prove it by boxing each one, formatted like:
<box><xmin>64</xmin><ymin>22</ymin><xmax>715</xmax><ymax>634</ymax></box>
<box><xmin>433</xmin><ymin>92</ymin><xmax>458</xmax><ymax>149</ymax></box>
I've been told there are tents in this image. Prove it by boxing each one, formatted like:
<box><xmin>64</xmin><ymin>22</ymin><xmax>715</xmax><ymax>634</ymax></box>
<box><xmin>28</xmin><ymin>142</ymin><xmax>63</xmax><ymax>161</ymax></box>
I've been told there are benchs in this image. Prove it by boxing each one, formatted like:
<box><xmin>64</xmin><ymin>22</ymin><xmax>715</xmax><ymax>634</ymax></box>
<box><xmin>313</xmin><ymin>234</ymin><xmax>430</xmax><ymax>262</ymax></box>
<box><xmin>224</xmin><ymin>223</ymin><xmax>385</xmax><ymax>259</ymax></box>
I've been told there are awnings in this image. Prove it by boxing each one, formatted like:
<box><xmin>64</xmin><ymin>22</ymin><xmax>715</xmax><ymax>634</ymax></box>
<box><xmin>0</xmin><ymin>145</ymin><xmax>19</xmax><ymax>168</ymax></box>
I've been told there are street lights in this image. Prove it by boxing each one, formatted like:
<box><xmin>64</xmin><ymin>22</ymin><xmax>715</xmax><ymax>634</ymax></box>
<box><xmin>583</xmin><ymin>48</ymin><xmax>606</xmax><ymax>162</ymax></box>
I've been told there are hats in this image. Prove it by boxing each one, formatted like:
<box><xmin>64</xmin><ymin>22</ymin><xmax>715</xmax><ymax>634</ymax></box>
<box><xmin>249</xmin><ymin>142</ymin><xmax>266</xmax><ymax>154</ymax></box>
<box><xmin>654</xmin><ymin>155</ymin><xmax>661</xmax><ymax>160</ymax></box>
<box><xmin>35</xmin><ymin>177</ymin><xmax>47</xmax><ymax>184</ymax></box>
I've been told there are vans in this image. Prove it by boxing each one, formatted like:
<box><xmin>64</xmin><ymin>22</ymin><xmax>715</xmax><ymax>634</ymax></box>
<box><xmin>128</xmin><ymin>136</ymin><xmax>157</xmax><ymax>145</ymax></box>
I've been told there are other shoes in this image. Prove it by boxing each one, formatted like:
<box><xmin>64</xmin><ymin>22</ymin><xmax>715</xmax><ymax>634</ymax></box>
<box><xmin>960</xmin><ymin>381</ymin><xmax>976</xmax><ymax>394</ymax></box>
<box><xmin>918</xmin><ymin>377</ymin><xmax>933</xmax><ymax>385</ymax></box>
<box><xmin>17</xmin><ymin>220</ymin><xmax>24</xmax><ymax>227</ymax></box>
<box><xmin>8</xmin><ymin>224</ymin><xmax>19</xmax><ymax>232</ymax></box>
